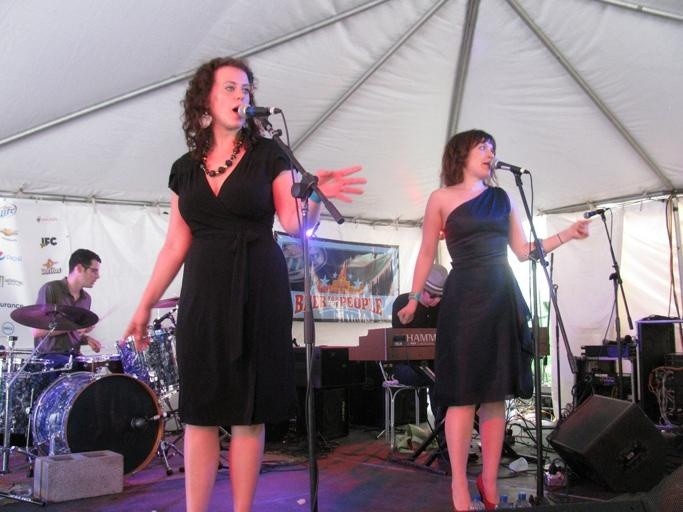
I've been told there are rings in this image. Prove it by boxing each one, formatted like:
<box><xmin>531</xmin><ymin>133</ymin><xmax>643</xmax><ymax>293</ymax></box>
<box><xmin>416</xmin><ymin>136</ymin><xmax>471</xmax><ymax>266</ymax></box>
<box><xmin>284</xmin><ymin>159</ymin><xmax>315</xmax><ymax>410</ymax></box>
<box><xmin>141</xmin><ymin>335</ymin><xmax>146</xmax><ymax>339</ymax></box>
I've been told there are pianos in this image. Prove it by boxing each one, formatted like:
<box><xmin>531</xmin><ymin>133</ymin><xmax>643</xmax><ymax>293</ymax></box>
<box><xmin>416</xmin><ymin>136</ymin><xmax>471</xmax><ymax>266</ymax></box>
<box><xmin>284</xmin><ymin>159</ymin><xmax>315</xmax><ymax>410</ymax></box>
<box><xmin>348</xmin><ymin>326</ymin><xmax>551</xmax><ymax>363</ymax></box>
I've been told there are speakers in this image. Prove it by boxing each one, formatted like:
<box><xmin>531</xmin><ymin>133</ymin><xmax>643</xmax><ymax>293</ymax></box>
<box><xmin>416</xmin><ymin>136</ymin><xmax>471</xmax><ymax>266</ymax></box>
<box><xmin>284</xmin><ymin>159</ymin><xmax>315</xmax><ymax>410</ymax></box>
<box><xmin>465</xmin><ymin>498</ymin><xmax>651</xmax><ymax>512</ymax></box>
<box><xmin>546</xmin><ymin>393</ymin><xmax>669</xmax><ymax>495</ymax></box>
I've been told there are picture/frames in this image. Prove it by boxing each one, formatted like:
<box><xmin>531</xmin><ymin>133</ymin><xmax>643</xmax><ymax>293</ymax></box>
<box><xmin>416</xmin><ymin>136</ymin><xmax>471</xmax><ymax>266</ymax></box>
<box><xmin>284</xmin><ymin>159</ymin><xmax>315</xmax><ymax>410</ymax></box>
<box><xmin>273</xmin><ymin>230</ymin><xmax>402</xmax><ymax>322</ymax></box>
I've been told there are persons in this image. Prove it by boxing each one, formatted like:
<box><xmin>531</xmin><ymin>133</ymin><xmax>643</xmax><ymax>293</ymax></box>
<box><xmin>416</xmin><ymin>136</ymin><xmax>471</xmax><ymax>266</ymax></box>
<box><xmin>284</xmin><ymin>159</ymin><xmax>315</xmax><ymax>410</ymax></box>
<box><xmin>116</xmin><ymin>55</ymin><xmax>364</xmax><ymax>510</ymax></box>
<box><xmin>398</xmin><ymin>128</ymin><xmax>589</xmax><ymax>512</ymax></box>
<box><xmin>28</xmin><ymin>248</ymin><xmax>103</xmax><ymax>366</ymax></box>
<box><xmin>385</xmin><ymin>263</ymin><xmax>480</xmax><ymax>463</ymax></box>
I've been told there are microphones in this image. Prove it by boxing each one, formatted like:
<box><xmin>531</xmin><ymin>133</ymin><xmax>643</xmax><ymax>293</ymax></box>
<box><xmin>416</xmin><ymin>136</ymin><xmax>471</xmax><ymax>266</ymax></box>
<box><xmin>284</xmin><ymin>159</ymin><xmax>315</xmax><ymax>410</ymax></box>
<box><xmin>583</xmin><ymin>206</ymin><xmax>610</xmax><ymax>219</ymax></box>
<box><xmin>237</xmin><ymin>103</ymin><xmax>280</xmax><ymax>118</ymax></box>
<box><xmin>489</xmin><ymin>158</ymin><xmax>527</xmax><ymax>174</ymax></box>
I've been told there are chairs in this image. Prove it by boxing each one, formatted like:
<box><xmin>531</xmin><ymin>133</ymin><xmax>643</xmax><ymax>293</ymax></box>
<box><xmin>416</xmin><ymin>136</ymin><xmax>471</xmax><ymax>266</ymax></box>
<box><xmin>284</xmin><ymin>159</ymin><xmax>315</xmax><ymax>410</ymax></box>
<box><xmin>379</xmin><ymin>362</ymin><xmax>427</xmax><ymax>450</ymax></box>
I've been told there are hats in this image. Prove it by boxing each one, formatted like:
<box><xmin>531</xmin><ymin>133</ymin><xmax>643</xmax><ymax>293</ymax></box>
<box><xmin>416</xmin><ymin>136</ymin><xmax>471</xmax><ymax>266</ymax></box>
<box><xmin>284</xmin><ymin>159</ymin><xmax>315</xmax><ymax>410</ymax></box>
<box><xmin>423</xmin><ymin>263</ymin><xmax>449</xmax><ymax>296</ymax></box>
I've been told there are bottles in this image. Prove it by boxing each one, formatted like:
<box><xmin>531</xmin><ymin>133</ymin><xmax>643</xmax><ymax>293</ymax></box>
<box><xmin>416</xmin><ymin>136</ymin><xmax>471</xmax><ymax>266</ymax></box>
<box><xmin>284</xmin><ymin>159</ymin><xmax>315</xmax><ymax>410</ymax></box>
<box><xmin>467</xmin><ymin>492</ymin><xmax>531</xmax><ymax>512</ymax></box>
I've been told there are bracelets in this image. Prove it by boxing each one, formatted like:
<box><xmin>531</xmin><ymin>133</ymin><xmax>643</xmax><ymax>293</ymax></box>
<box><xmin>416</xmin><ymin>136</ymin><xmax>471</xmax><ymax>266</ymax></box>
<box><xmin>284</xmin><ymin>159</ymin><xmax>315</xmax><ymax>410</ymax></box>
<box><xmin>557</xmin><ymin>233</ymin><xmax>564</xmax><ymax>246</ymax></box>
<box><xmin>405</xmin><ymin>291</ymin><xmax>422</xmax><ymax>303</ymax></box>
<box><xmin>307</xmin><ymin>189</ymin><xmax>324</xmax><ymax>208</ymax></box>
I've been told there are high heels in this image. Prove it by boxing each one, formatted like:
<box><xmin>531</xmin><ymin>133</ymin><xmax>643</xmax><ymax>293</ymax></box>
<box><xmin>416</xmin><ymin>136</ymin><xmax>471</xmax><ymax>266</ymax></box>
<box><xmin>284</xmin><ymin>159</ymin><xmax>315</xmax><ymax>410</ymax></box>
<box><xmin>474</xmin><ymin>468</ymin><xmax>503</xmax><ymax>510</ymax></box>
<box><xmin>446</xmin><ymin>475</ymin><xmax>476</xmax><ymax>512</ymax></box>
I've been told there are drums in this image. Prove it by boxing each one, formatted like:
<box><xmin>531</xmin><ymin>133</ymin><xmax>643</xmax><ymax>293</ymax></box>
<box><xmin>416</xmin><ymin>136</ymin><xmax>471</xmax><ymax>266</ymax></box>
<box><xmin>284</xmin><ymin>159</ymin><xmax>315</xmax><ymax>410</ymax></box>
<box><xmin>75</xmin><ymin>354</ymin><xmax>124</xmax><ymax>374</ymax></box>
<box><xmin>32</xmin><ymin>371</ymin><xmax>165</xmax><ymax>477</ymax></box>
<box><xmin>116</xmin><ymin>327</ymin><xmax>180</xmax><ymax>400</ymax></box>
<box><xmin>0</xmin><ymin>369</ymin><xmax>68</xmax><ymax>435</ymax></box>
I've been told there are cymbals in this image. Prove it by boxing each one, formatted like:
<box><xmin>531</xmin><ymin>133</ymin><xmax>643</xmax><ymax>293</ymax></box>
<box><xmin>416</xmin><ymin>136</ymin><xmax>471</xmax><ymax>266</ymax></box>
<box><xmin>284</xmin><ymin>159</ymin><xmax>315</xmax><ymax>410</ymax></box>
<box><xmin>152</xmin><ymin>297</ymin><xmax>179</xmax><ymax>308</ymax></box>
<box><xmin>10</xmin><ymin>303</ymin><xmax>99</xmax><ymax>330</ymax></box>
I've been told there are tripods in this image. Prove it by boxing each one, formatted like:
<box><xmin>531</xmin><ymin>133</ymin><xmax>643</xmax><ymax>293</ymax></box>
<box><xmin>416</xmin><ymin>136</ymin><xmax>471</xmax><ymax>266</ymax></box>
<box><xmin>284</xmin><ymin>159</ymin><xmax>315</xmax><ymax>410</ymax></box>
<box><xmin>1</xmin><ymin>356</ymin><xmax>40</xmax><ymax>474</ymax></box>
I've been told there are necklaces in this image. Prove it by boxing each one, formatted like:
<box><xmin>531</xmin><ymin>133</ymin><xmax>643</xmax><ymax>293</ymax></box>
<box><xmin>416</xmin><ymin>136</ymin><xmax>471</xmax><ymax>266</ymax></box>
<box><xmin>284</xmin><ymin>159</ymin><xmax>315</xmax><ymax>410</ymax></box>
<box><xmin>192</xmin><ymin>133</ymin><xmax>248</xmax><ymax>177</ymax></box>
